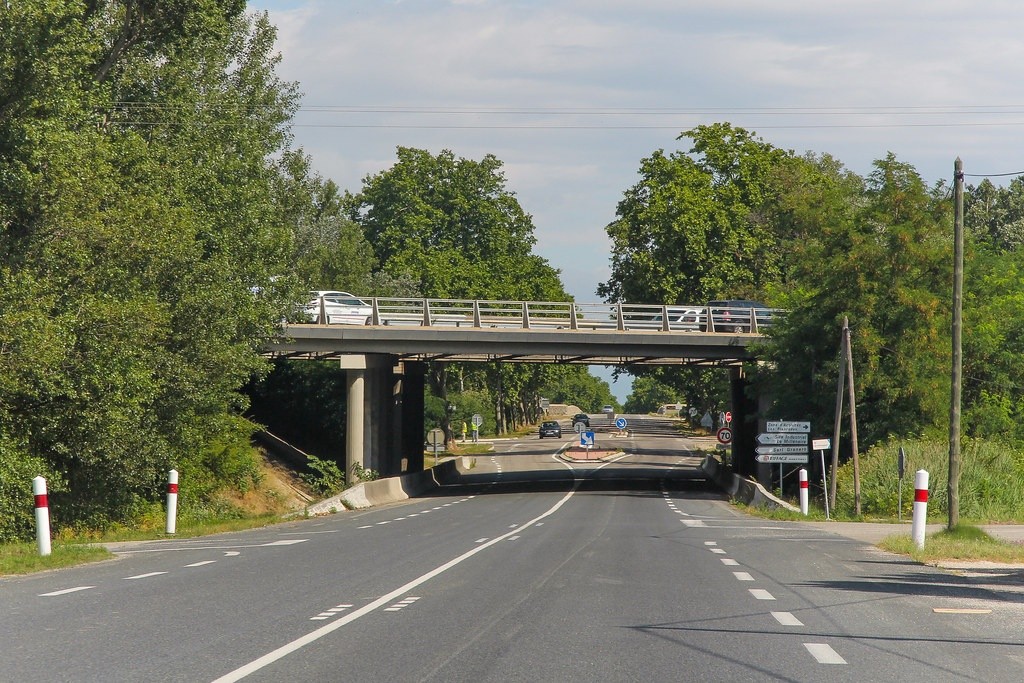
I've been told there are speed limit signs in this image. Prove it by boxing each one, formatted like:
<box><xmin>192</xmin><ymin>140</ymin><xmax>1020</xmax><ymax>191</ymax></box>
<box><xmin>716</xmin><ymin>427</ymin><xmax>734</xmax><ymax>444</ymax></box>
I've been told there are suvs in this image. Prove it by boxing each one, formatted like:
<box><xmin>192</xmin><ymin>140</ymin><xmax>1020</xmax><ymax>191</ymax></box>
<box><xmin>697</xmin><ymin>299</ymin><xmax>782</xmax><ymax>335</ymax></box>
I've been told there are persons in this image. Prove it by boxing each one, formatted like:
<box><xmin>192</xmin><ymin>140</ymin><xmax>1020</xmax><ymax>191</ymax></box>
<box><xmin>470</xmin><ymin>421</ymin><xmax>478</xmax><ymax>443</ymax></box>
<box><xmin>461</xmin><ymin>419</ymin><xmax>467</xmax><ymax>443</ymax></box>
<box><xmin>447</xmin><ymin>423</ymin><xmax>454</xmax><ymax>443</ymax></box>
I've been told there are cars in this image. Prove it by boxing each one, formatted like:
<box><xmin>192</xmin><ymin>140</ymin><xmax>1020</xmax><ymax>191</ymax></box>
<box><xmin>538</xmin><ymin>420</ymin><xmax>562</xmax><ymax>437</ymax></box>
<box><xmin>602</xmin><ymin>404</ymin><xmax>615</xmax><ymax>413</ymax></box>
<box><xmin>293</xmin><ymin>289</ymin><xmax>380</xmax><ymax>327</ymax></box>
<box><xmin>570</xmin><ymin>412</ymin><xmax>590</xmax><ymax>427</ymax></box>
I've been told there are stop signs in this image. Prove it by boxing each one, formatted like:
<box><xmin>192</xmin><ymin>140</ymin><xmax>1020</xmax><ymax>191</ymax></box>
<box><xmin>725</xmin><ymin>412</ymin><xmax>732</xmax><ymax>422</ymax></box>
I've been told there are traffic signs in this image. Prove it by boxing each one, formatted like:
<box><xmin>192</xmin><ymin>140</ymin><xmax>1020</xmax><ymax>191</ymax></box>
<box><xmin>766</xmin><ymin>421</ymin><xmax>811</xmax><ymax>434</ymax></box>
<box><xmin>754</xmin><ymin>445</ymin><xmax>810</xmax><ymax>456</ymax></box>
<box><xmin>755</xmin><ymin>433</ymin><xmax>809</xmax><ymax>445</ymax></box>
<box><xmin>754</xmin><ymin>454</ymin><xmax>809</xmax><ymax>465</ymax></box>
